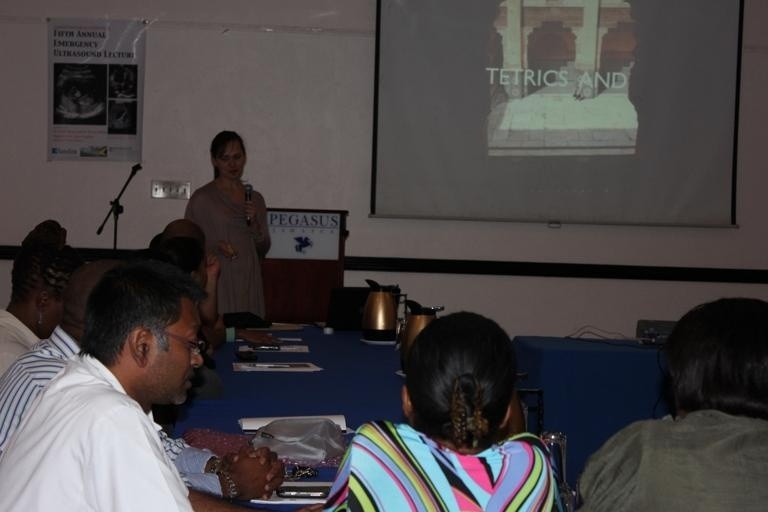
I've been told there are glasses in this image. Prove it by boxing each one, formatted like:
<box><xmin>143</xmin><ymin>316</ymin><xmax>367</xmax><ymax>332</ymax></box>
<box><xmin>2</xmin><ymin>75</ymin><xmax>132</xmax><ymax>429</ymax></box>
<box><xmin>163</xmin><ymin>331</ymin><xmax>205</xmax><ymax>355</ymax></box>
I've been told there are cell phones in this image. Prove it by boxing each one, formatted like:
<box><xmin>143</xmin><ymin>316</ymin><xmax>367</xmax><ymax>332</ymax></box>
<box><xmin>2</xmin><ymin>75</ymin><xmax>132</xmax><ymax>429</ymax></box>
<box><xmin>254</xmin><ymin>343</ymin><xmax>280</xmax><ymax>351</ymax></box>
<box><xmin>279</xmin><ymin>486</ymin><xmax>331</xmax><ymax>498</ymax></box>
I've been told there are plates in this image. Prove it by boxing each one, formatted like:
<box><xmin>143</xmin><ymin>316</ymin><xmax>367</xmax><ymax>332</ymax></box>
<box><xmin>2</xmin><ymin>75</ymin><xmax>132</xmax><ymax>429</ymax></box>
<box><xmin>359</xmin><ymin>338</ymin><xmax>397</xmax><ymax>347</ymax></box>
<box><xmin>394</xmin><ymin>370</ymin><xmax>408</xmax><ymax>379</ymax></box>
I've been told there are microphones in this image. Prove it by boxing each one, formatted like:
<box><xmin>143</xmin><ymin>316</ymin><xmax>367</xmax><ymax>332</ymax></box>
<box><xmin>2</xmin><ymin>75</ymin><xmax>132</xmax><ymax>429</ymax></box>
<box><xmin>244</xmin><ymin>183</ymin><xmax>253</xmax><ymax>223</ymax></box>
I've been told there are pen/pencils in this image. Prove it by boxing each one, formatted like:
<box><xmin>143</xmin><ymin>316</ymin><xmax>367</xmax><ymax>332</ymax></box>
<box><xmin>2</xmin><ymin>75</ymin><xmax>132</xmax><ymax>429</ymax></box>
<box><xmin>248</xmin><ymin>364</ymin><xmax>291</xmax><ymax>368</ymax></box>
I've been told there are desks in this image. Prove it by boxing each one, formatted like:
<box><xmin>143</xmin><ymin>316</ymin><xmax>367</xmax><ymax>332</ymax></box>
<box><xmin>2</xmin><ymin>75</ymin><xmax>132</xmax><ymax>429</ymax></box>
<box><xmin>512</xmin><ymin>334</ymin><xmax>666</xmax><ymax>493</ymax></box>
<box><xmin>168</xmin><ymin>321</ymin><xmax>406</xmax><ymax>511</ymax></box>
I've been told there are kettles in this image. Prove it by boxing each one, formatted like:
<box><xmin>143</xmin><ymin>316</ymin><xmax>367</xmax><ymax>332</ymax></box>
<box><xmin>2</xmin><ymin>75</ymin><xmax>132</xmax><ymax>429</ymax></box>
<box><xmin>361</xmin><ymin>279</ymin><xmax>402</xmax><ymax>341</ymax></box>
<box><xmin>400</xmin><ymin>299</ymin><xmax>445</xmax><ymax>374</ymax></box>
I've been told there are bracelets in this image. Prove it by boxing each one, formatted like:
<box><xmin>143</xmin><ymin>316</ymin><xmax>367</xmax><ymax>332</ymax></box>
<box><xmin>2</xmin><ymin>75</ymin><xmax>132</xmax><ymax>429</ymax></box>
<box><xmin>218</xmin><ymin>468</ymin><xmax>240</xmax><ymax>501</ymax></box>
<box><xmin>208</xmin><ymin>457</ymin><xmax>220</xmax><ymax>473</ymax></box>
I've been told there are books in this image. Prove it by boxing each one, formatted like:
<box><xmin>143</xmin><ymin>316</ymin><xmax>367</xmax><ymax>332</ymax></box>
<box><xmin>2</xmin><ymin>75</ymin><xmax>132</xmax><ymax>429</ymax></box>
<box><xmin>249</xmin><ymin>463</ymin><xmax>339</xmax><ymax>506</ymax></box>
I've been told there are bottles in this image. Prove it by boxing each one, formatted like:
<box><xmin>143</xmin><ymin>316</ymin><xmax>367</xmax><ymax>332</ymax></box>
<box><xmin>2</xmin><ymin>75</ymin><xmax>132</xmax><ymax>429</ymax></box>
<box><xmin>539</xmin><ymin>431</ymin><xmax>573</xmax><ymax>512</ymax></box>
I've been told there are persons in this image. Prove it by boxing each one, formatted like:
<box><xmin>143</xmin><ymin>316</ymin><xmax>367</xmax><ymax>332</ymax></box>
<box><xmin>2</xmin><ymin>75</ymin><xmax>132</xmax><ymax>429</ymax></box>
<box><xmin>1</xmin><ymin>241</ymin><xmax>87</xmax><ymax>376</ymax></box>
<box><xmin>140</xmin><ymin>235</ymin><xmax>225</xmax><ymax>401</ymax></box>
<box><xmin>149</xmin><ymin>219</ymin><xmax>281</xmax><ymax>346</ymax></box>
<box><xmin>1</xmin><ymin>261</ymin><xmax>285</xmax><ymax>501</ymax></box>
<box><xmin>575</xmin><ymin>296</ymin><xmax>768</xmax><ymax>512</ymax></box>
<box><xmin>184</xmin><ymin>129</ymin><xmax>271</xmax><ymax>329</ymax></box>
<box><xmin>322</xmin><ymin>307</ymin><xmax>565</xmax><ymax>512</ymax></box>
<box><xmin>1</xmin><ymin>261</ymin><xmax>324</xmax><ymax>511</ymax></box>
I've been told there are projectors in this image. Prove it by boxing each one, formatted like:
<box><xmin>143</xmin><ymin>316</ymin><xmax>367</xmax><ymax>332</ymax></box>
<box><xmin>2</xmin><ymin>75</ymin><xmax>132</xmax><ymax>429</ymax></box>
<box><xmin>635</xmin><ymin>319</ymin><xmax>677</xmax><ymax>345</ymax></box>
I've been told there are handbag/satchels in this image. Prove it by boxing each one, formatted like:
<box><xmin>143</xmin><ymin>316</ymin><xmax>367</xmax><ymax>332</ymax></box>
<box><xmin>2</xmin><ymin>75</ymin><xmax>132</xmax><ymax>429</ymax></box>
<box><xmin>249</xmin><ymin>417</ymin><xmax>347</xmax><ymax>467</ymax></box>
